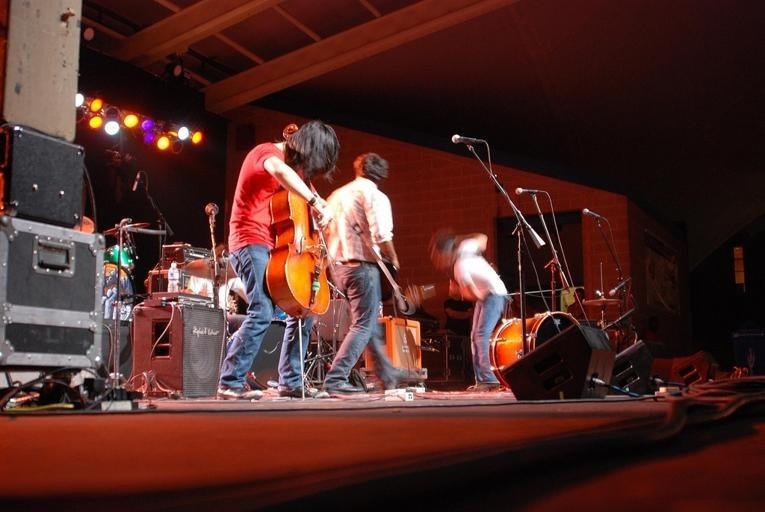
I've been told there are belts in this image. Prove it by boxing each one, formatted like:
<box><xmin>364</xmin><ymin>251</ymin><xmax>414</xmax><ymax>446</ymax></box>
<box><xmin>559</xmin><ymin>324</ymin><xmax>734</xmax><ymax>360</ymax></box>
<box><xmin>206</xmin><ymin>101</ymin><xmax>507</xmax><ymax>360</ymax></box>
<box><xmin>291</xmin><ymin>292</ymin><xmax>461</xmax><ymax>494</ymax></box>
<box><xmin>342</xmin><ymin>260</ymin><xmax>379</xmax><ymax>267</ymax></box>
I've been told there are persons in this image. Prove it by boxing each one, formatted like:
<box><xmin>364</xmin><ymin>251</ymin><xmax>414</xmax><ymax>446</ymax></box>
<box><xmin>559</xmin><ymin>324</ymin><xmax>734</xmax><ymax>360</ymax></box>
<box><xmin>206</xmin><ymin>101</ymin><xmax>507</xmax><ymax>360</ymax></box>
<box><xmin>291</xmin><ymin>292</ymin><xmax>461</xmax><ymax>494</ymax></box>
<box><xmin>566</xmin><ymin>288</ymin><xmax>587</xmax><ymax>318</ymax></box>
<box><xmin>428</xmin><ymin>230</ymin><xmax>532</xmax><ymax>392</ymax></box>
<box><xmin>216</xmin><ymin>122</ymin><xmax>340</xmax><ymax>400</ymax></box>
<box><xmin>323</xmin><ymin>154</ymin><xmax>409</xmax><ymax>393</ymax></box>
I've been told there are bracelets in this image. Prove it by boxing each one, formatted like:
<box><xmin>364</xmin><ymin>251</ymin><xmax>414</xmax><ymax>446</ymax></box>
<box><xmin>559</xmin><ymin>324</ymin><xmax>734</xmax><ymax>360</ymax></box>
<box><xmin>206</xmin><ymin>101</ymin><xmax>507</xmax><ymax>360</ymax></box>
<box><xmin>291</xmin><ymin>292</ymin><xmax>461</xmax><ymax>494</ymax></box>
<box><xmin>307</xmin><ymin>196</ymin><xmax>318</xmax><ymax>205</ymax></box>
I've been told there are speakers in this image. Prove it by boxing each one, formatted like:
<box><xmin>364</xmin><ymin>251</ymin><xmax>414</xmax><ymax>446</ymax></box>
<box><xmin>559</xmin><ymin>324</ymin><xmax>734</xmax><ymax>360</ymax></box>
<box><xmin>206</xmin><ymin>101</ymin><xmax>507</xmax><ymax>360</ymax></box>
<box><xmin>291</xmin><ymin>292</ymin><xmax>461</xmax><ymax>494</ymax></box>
<box><xmin>501</xmin><ymin>325</ymin><xmax>616</xmax><ymax>401</ymax></box>
<box><xmin>133</xmin><ymin>304</ymin><xmax>222</xmax><ymax>399</ymax></box>
<box><xmin>609</xmin><ymin>338</ymin><xmax>653</xmax><ymax>396</ymax></box>
<box><xmin>657</xmin><ymin>340</ymin><xmax>727</xmax><ymax>385</ymax></box>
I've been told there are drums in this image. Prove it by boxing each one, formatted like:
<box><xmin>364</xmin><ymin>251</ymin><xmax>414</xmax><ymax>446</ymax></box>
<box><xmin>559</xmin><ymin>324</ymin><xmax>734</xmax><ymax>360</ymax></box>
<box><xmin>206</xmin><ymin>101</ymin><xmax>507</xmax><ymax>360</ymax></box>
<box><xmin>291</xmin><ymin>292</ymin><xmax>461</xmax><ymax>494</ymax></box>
<box><xmin>490</xmin><ymin>311</ymin><xmax>581</xmax><ymax>390</ymax></box>
<box><xmin>104</xmin><ymin>243</ymin><xmax>134</xmax><ymax>274</ymax></box>
<box><xmin>103</xmin><ymin>262</ymin><xmax>137</xmax><ymax>320</ymax></box>
<box><xmin>225</xmin><ymin>307</ymin><xmax>310</xmax><ymax>390</ymax></box>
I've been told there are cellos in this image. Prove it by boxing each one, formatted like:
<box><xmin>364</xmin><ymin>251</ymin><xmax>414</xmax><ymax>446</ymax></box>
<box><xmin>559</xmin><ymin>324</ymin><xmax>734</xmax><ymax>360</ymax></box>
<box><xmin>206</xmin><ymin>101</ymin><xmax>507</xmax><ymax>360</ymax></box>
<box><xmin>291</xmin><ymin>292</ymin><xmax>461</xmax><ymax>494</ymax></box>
<box><xmin>266</xmin><ymin>124</ymin><xmax>330</xmax><ymax>319</ymax></box>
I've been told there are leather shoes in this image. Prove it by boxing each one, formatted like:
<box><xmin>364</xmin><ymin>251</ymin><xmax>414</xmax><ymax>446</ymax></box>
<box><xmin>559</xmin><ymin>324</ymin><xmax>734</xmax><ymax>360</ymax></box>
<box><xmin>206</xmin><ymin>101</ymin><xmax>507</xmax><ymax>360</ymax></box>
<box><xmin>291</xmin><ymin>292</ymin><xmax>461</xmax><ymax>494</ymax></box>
<box><xmin>323</xmin><ymin>383</ymin><xmax>364</xmax><ymax>395</ymax></box>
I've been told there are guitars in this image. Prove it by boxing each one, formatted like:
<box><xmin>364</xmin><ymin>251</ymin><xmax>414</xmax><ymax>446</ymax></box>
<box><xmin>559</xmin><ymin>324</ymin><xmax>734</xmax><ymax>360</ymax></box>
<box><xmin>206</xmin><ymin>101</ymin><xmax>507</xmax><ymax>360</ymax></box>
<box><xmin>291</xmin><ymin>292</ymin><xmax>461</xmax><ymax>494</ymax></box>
<box><xmin>380</xmin><ymin>258</ymin><xmax>398</xmax><ymax>301</ymax></box>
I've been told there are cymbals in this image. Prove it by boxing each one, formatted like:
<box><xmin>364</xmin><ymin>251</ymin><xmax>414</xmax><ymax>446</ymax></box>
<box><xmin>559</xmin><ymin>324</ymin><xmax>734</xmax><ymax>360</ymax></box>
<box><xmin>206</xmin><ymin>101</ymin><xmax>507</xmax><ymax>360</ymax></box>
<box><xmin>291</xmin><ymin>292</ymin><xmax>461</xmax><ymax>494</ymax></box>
<box><xmin>103</xmin><ymin>222</ymin><xmax>149</xmax><ymax>236</ymax></box>
<box><xmin>583</xmin><ymin>298</ymin><xmax>622</xmax><ymax>305</ymax></box>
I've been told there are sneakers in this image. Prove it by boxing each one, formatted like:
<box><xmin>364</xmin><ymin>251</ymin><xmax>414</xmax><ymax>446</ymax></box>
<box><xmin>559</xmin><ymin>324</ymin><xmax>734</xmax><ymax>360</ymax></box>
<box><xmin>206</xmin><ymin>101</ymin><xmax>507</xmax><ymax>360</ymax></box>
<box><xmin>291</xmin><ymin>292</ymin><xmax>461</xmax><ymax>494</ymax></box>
<box><xmin>216</xmin><ymin>383</ymin><xmax>263</xmax><ymax>401</ymax></box>
<box><xmin>467</xmin><ymin>382</ymin><xmax>499</xmax><ymax>392</ymax></box>
<box><xmin>280</xmin><ymin>386</ymin><xmax>327</xmax><ymax>397</ymax></box>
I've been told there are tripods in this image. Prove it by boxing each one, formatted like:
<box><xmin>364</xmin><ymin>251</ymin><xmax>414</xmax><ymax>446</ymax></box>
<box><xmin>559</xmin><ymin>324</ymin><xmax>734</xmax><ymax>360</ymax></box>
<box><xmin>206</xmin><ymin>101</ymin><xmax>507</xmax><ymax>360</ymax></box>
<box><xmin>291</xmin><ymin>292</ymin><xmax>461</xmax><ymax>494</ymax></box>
<box><xmin>305</xmin><ymin>324</ymin><xmax>330</xmax><ymax>386</ymax></box>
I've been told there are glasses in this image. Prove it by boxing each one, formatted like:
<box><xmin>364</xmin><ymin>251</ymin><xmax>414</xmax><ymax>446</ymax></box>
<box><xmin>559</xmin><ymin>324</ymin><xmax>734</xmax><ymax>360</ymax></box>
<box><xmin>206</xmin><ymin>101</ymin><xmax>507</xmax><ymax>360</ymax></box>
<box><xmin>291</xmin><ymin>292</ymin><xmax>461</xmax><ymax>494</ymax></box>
<box><xmin>573</xmin><ymin>294</ymin><xmax>581</xmax><ymax>297</ymax></box>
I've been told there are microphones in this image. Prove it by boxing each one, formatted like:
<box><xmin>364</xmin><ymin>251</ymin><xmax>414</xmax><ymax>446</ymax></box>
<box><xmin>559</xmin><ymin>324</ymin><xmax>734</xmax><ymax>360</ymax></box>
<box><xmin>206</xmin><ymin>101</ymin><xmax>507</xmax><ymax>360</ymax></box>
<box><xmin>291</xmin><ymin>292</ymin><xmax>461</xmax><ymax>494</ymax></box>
<box><xmin>205</xmin><ymin>204</ymin><xmax>218</xmax><ymax>216</ymax></box>
<box><xmin>609</xmin><ymin>277</ymin><xmax>629</xmax><ymax>296</ymax></box>
<box><xmin>452</xmin><ymin>135</ymin><xmax>482</xmax><ymax>145</ymax></box>
<box><xmin>583</xmin><ymin>208</ymin><xmax>602</xmax><ymax>219</ymax></box>
<box><xmin>132</xmin><ymin>172</ymin><xmax>143</xmax><ymax>191</ymax></box>
<box><xmin>515</xmin><ymin>186</ymin><xmax>539</xmax><ymax>195</ymax></box>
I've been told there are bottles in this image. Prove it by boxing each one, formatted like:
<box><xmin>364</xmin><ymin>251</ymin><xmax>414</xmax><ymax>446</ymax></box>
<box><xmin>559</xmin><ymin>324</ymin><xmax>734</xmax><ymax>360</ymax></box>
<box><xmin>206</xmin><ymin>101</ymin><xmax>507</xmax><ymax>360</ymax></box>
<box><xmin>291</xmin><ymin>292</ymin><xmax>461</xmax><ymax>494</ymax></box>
<box><xmin>113</xmin><ymin>301</ymin><xmax>132</xmax><ymax>321</ymax></box>
<box><xmin>167</xmin><ymin>261</ymin><xmax>180</xmax><ymax>293</ymax></box>
